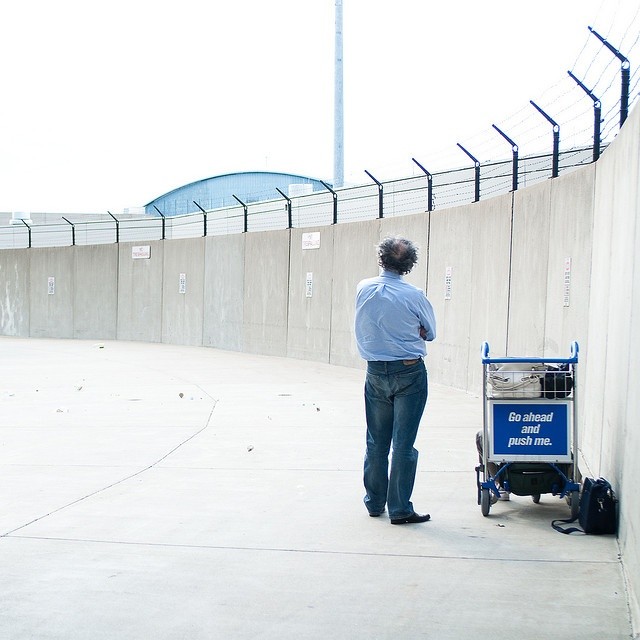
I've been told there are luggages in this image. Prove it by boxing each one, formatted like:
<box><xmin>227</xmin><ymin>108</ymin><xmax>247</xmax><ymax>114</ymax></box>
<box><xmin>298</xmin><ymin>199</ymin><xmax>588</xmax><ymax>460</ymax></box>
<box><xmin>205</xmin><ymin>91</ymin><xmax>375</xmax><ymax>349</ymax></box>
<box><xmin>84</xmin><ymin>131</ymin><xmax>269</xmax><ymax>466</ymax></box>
<box><xmin>499</xmin><ymin>462</ymin><xmax>573</xmax><ymax>496</ymax></box>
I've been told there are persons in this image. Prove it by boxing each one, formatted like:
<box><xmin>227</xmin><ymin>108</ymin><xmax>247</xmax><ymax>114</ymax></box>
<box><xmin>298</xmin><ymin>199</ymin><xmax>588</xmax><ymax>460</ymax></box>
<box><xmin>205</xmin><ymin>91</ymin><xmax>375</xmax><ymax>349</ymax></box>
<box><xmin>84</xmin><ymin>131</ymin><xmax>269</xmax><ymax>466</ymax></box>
<box><xmin>354</xmin><ymin>237</ymin><xmax>436</xmax><ymax>527</ymax></box>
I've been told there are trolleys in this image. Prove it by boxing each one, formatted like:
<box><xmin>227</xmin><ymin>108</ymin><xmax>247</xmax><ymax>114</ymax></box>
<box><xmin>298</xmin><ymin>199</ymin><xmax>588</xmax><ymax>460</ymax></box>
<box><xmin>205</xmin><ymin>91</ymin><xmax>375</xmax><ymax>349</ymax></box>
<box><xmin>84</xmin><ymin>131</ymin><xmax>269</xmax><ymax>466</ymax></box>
<box><xmin>481</xmin><ymin>342</ymin><xmax>581</xmax><ymax>519</ymax></box>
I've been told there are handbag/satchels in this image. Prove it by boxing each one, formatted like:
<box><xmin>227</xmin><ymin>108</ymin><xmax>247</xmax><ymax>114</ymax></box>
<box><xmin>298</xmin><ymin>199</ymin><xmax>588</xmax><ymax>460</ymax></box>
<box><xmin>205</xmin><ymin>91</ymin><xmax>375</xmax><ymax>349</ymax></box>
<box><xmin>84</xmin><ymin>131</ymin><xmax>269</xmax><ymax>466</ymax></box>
<box><xmin>540</xmin><ymin>363</ymin><xmax>574</xmax><ymax>399</ymax></box>
<box><xmin>487</xmin><ymin>363</ymin><xmax>546</xmax><ymax>398</ymax></box>
<box><xmin>551</xmin><ymin>477</ymin><xmax>618</xmax><ymax>535</ymax></box>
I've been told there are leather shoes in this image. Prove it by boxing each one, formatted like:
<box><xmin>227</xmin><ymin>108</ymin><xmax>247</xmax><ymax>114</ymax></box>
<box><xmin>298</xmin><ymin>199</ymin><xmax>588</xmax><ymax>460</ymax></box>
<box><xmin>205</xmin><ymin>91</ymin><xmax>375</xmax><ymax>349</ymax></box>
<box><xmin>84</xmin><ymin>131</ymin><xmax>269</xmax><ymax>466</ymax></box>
<box><xmin>391</xmin><ymin>513</ymin><xmax>430</xmax><ymax>525</ymax></box>
